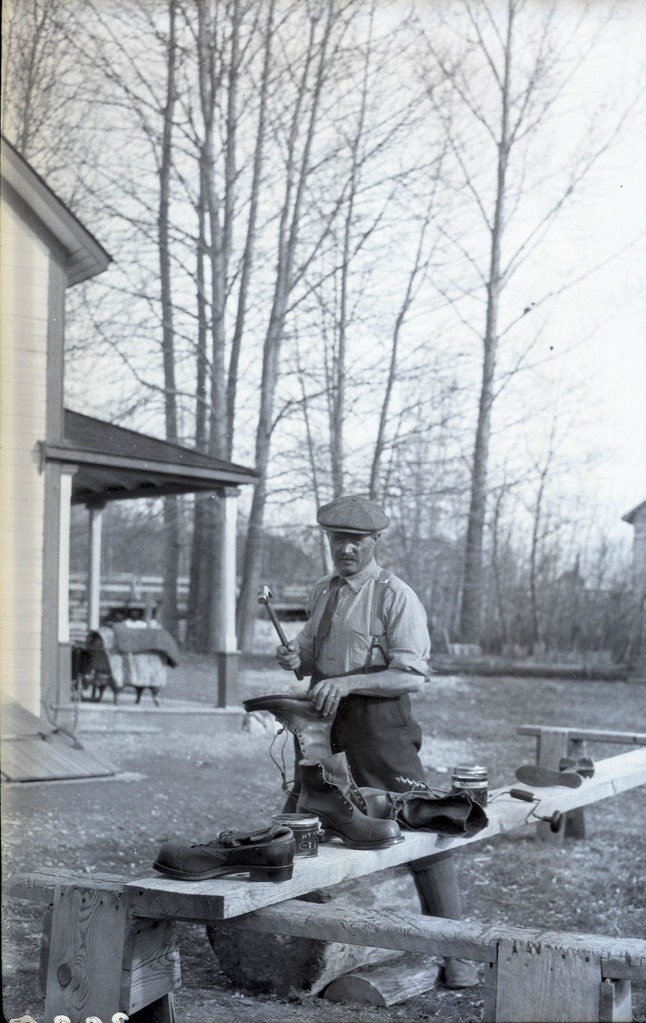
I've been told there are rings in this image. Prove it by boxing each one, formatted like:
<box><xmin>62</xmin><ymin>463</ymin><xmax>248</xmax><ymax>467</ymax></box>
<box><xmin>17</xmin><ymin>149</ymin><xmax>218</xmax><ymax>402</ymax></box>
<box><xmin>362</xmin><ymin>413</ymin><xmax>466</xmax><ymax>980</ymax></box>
<box><xmin>328</xmin><ymin>693</ymin><xmax>334</xmax><ymax>696</ymax></box>
<box><xmin>335</xmin><ymin>697</ymin><xmax>340</xmax><ymax>701</ymax></box>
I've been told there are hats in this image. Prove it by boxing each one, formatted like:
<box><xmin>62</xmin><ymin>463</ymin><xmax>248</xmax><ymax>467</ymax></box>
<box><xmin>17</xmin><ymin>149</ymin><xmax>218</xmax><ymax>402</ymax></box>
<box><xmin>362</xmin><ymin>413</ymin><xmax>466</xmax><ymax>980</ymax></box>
<box><xmin>317</xmin><ymin>496</ymin><xmax>389</xmax><ymax>535</ymax></box>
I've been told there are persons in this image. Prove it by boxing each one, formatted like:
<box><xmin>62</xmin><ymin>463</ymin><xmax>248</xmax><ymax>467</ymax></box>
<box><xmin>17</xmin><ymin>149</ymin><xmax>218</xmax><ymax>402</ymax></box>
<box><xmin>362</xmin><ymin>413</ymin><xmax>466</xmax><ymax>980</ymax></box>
<box><xmin>275</xmin><ymin>498</ymin><xmax>480</xmax><ymax>987</ymax></box>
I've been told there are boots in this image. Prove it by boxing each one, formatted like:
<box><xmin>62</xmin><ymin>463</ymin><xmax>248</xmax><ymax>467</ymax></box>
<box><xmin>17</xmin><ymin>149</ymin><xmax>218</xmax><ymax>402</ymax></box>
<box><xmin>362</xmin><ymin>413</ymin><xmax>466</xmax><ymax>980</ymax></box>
<box><xmin>243</xmin><ymin>693</ymin><xmax>338</xmax><ymax>760</ymax></box>
<box><xmin>297</xmin><ymin>752</ymin><xmax>405</xmax><ymax>849</ymax></box>
<box><xmin>356</xmin><ymin>781</ymin><xmax>488</xmax><ymax>840</ymax></box>
<box><xmin>413</xmin><ymin>859</ymin><xmax>479</xmax><ymax>988</ymax></box>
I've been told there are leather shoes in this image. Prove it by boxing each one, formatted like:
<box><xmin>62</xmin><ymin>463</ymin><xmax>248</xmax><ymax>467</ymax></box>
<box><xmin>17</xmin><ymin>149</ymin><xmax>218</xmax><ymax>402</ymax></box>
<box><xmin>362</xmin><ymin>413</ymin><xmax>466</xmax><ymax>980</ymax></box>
<box><xmin>153</xmin><ymin>825</ymin><xmax>296</xmax><ymax>882</ymax></box>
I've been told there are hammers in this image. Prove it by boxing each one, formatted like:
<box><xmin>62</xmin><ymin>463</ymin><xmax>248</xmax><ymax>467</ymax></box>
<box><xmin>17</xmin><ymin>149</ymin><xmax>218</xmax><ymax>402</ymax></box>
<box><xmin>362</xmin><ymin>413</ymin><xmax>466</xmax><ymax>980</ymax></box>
<box><xmin>258</xmin><ymin>586</ymin><xmax>304</xmax><ymax>680</ymax></box>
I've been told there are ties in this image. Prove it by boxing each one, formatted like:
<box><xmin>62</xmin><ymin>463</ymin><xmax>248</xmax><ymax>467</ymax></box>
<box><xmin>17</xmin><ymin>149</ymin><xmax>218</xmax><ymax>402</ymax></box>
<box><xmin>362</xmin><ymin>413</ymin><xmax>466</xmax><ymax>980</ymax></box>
<box><xmin>313</xmin><ymin>579</ymin><xmax>346</xmax><ymax>661</ymax></box>
